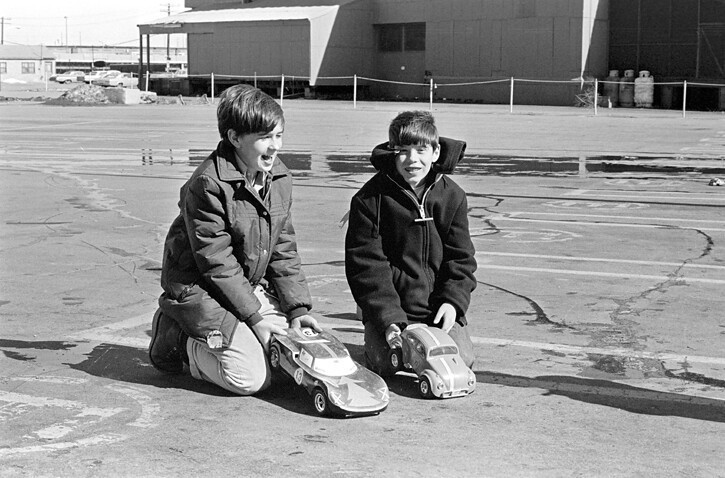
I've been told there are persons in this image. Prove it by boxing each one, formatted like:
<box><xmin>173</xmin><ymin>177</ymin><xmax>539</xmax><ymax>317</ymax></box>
<box><xmin>344</xmin><ymin>109</ymin><xmax>477</xmax><ymax>382</ymax></box>
<box><xmin>148</xmin><ymin>83</ymin><xmax>322</xmax><ymax>396</ymax></box>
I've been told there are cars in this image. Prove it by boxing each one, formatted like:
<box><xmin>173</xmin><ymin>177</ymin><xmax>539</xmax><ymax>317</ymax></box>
<box><xmin>268</xmin><ymin>326</ymin><xmax>390</xmax><ymax>418</ymax></box>
<box><xmin>53</xmin><ymin>69</ymin><xmax>140</xmax><ymax>89</ymax></box>
<box><xmin>387</xmin><ymin>323</ymin><xmax>477</xmax><ymax>399</ymax></box>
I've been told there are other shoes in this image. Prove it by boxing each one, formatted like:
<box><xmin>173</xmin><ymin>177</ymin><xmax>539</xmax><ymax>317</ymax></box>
<box><xmin>148</xmin><ymin>308</ymin><xmax>186</xmax><ymax>375</ymax></box>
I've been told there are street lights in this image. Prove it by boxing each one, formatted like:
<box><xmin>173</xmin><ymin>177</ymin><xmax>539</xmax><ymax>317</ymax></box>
<box><xmin>63</xmin><ymin>16</ymin><xmax>68</xmax><ymax>49</ymax></box>
